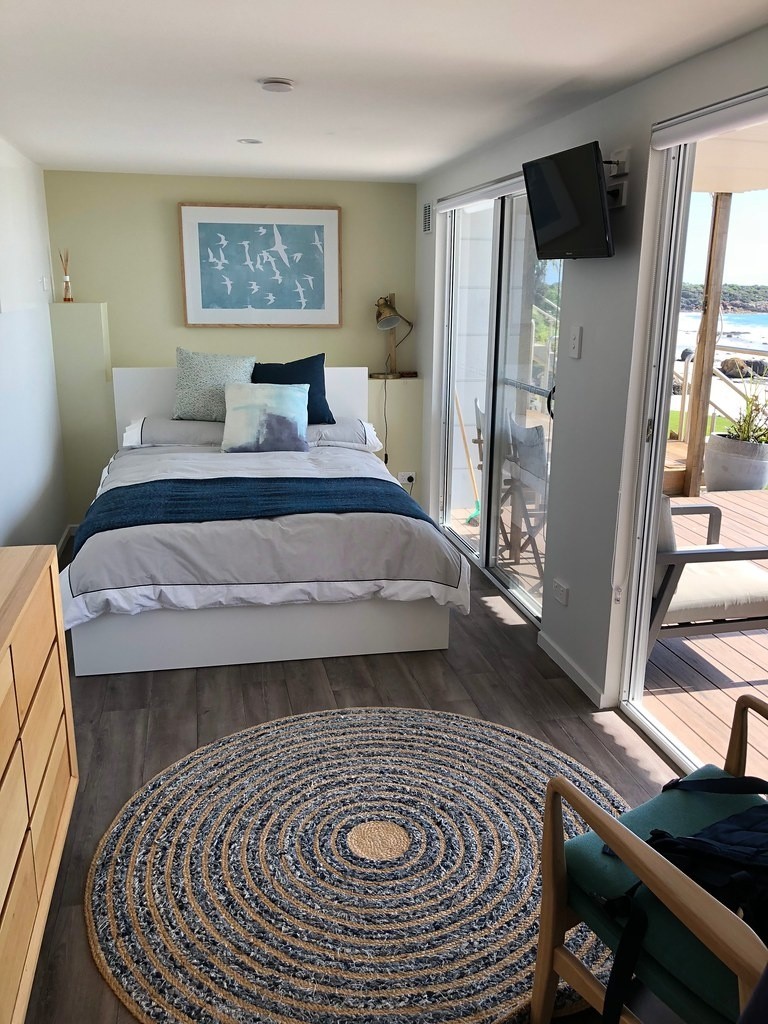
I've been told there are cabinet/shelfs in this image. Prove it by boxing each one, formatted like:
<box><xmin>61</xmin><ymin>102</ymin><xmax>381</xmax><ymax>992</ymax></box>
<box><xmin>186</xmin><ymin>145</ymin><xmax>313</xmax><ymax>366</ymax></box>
<box><xmin>0</xmin><ymin>544</ymin><xmax>80</xmax><ymax>1024</ymax></box>
<box><xmin>57</xmin><ymin>367</ymin><xmax>471</xmax><ymax>677</ymax></box>
<box><xmin>368</xmin><ymin>378</ymin><xmax>423</xmax><ymax>507</ymax></box>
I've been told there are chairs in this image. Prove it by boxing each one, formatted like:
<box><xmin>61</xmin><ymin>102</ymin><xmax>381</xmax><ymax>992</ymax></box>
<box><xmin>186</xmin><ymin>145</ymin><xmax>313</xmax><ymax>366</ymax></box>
<box><xmin>474</xmin><ymin>397</ymin><xmax>552</xmax><ymax>546</ymax></box>
<box><xmin>510</xmin><ymin>411</ymin><xmax>551</xmax><ymax>581</ymax></box>
<box><xmin>646</xmin><ymin>493</ymin><xmax>768</xmax><ymax>664</ymax></box>
<box><xmin>532</xmin><ymin>694</ymin><xmax>768</xmax><ymax>1024</ymax></box>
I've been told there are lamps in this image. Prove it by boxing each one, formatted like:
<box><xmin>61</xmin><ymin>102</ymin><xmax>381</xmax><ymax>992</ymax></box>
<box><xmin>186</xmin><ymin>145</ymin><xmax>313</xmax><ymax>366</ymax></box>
<box><xmin>371</xmin><ymin>292</ymin><xmax>416</xmax><ymax>378</ymax></box>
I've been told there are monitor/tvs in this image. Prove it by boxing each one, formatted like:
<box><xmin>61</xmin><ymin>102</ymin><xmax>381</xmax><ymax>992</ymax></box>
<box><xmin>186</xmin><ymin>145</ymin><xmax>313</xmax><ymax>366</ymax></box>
<box><xmin>522</xmin><ymin>140</ymin><xmax>614</xmax><ymax>260</ymax></box>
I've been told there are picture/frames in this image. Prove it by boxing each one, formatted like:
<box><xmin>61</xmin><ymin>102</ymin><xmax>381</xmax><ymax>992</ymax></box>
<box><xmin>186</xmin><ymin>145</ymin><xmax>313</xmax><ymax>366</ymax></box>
<box><xmin>178</xmin><ymin>202</ymin><xmax>343</xmax><ymax>329</ymax></box>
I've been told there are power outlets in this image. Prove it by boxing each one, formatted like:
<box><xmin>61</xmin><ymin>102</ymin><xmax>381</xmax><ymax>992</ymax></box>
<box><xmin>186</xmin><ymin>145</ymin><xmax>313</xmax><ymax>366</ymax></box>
<box><xmin>606</xmin><ymin>181</ymin><xmax>629</xmax><ymax>210</ymax></box>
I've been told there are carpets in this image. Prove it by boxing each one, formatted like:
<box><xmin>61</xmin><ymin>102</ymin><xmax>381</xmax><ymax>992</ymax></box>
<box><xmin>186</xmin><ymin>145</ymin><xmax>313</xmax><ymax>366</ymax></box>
<box><xmin>85</xmin><ymin>706</ymin><xmax>634</xmax><ymax>1024</ymax></box>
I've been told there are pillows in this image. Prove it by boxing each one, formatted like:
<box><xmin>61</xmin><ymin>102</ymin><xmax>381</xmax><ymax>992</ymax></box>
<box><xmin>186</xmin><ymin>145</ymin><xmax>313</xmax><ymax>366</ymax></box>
<box><xmin>221</xmin><ymin>381</ymin><xmax>310</xmax><ymax>451</ymax></box>
<box><xmin>122</xmin><ymin>415</ymin><xmax>384</xmax><ymax>453</ymax></box>
<box><xmin>169</xmin><ymin>346</ymin><xmax>255</xmax><ymax>422</ymax></box>
<box><xmin>398</xmin><ymin>472</ymin><xmax>416</xmax><ymax>483</ymax></box>
<box><xmin>251</xmin><ymin>353</ymin><xmax>336</xmax><ymax>425</ymax></box>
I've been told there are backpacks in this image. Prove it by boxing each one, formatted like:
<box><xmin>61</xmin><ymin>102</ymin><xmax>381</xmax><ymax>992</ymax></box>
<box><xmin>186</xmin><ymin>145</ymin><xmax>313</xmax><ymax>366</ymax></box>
<box><xmin>603</xmin><ymin>777</ymin><xmax>768</xmax><ymax>1024</ymax></box>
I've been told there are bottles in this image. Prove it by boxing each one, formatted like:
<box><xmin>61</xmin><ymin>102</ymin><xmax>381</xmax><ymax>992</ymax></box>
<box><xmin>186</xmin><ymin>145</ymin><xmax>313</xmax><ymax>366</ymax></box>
<box><xmin>63</xmin><ymin>276</ymin><xmax>73</xmax><ymax>302</ymax></box>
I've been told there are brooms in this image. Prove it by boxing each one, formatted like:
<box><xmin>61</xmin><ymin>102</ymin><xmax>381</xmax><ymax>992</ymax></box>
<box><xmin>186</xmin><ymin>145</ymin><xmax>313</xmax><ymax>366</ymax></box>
<box><xmin>454</xmin><ymin>386</ymin><xmax>481</xmax><ymax>528</ymax></box>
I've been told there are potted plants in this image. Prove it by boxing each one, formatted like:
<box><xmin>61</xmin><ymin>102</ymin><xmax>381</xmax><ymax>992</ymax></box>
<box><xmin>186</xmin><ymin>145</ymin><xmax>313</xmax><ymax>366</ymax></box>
<box><xmin>704</xmin><ymin>356</ymin><xmax>768</xmax><ymax>492</ymax></box>
<box><xmin>59</xmin><ymin>250</ymin><xmax>74</xmax><ymax>301</ymax></box>
